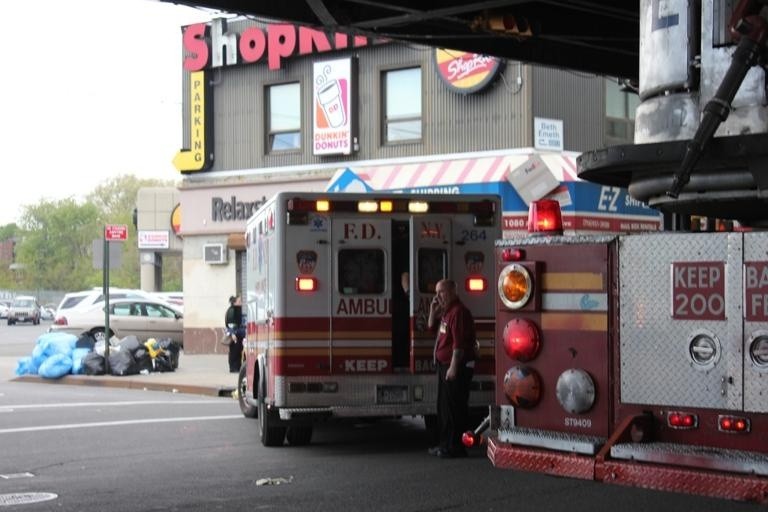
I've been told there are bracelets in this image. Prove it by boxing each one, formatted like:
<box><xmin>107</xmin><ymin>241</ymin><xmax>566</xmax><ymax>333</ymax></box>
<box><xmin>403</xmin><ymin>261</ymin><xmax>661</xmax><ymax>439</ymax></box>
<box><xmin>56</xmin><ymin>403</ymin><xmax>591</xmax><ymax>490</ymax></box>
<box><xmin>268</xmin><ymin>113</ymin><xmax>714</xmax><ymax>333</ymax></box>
<box><xmin>406</xmin><ymin>289</ymin><xmax>409</xmax><ymax>296</ymax></box>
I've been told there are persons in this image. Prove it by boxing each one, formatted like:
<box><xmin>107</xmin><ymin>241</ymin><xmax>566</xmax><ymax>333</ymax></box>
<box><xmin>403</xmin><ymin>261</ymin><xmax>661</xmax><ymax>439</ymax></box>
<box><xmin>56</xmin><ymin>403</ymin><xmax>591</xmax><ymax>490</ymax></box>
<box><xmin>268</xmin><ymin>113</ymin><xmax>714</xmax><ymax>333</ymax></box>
<box><xmin>225</xmin><ymin>295</ymin><xmax>247</xmax><ymax>374</ymax></box>
<box><xmin>399</xmin><ymin>269</ymin><xmax>410</xmax><ymax>298</ymax></box>
<box><xmin>426</xmin><ymin>280</ymin><xmax>478</xmax><ymax>455</ymax></box>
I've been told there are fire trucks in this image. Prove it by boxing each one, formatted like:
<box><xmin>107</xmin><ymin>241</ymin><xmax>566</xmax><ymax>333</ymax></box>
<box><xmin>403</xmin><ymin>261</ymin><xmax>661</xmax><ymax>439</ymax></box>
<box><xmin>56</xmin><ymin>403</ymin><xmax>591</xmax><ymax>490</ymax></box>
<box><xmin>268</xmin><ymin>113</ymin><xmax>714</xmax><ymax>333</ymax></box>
<box><xmin>237</xmin><ymin>187</ymin><xmax>501</xmax><ymax>450</ymax></box>
<box><xmin>488</xmin><ymin>198</ymin><xmax>768</xmax><ymax>509</ymax></box>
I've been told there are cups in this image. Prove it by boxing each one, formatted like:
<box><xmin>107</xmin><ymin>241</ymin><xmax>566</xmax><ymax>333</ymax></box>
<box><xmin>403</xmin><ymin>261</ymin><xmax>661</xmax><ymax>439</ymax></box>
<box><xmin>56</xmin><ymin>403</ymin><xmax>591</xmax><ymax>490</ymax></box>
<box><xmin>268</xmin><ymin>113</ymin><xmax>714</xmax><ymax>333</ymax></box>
<box><xmin>318</xmin><ymin>79</ymin><xmax>344</xmax><ymax>127</ymax></box>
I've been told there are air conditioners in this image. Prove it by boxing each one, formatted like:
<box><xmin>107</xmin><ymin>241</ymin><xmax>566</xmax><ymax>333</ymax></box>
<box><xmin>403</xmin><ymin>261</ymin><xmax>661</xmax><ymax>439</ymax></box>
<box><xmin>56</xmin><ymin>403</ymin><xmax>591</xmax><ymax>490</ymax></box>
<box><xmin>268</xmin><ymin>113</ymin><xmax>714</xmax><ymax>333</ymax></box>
<box><xmin>203</xmin><ymin>243</ymin><xmax>227</xmax><ymax>264</ymax></box>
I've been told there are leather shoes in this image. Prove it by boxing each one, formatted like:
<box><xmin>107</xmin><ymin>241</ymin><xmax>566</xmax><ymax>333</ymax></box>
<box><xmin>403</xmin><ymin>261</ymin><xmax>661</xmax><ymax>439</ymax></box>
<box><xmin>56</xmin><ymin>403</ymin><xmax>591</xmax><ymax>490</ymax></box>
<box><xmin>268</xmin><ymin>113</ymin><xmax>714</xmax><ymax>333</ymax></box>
<box><xmin>427</xmin><ymin>445</ymin><xmax>443</xmax><ymax>456</ymax></box>
<box><xmin>437</xmin><ymin>448</ymin><xmax>468</xmax><ymax>458</ymax></box>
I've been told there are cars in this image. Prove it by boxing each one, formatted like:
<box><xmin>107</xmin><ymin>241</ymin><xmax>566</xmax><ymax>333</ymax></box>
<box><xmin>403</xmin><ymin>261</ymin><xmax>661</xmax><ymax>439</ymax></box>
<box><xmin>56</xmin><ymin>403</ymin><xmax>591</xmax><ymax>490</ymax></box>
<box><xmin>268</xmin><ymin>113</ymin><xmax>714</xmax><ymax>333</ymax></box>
<box><xmin>0</xmin><ymin>286</ymin><xmax>184</xmax><ymax>351</ymax></box>
